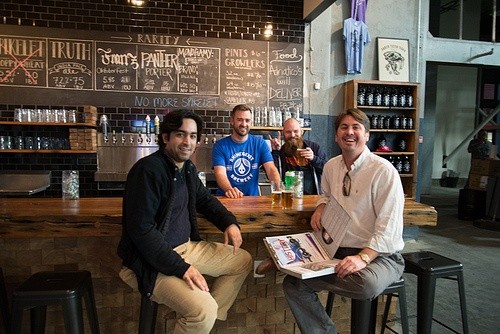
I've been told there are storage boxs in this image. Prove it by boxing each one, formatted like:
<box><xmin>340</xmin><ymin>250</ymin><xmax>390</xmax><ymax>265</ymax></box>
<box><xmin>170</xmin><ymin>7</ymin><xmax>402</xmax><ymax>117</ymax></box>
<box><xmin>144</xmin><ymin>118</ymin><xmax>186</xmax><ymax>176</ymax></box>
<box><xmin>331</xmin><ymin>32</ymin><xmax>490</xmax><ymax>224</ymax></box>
<box><xmin>471</xmin><ymin>158</ymin><xmax>500</xmax><ymax>175</ymax></box>
<box><xmin>69</xmin><ymin>104</ymin><xmax>99</xmax><ymax>152</ymax></box>
<box><xmin>470</xmin><ymin>175</ymin><xmax>488</xmax><ymax>191</ymax></box>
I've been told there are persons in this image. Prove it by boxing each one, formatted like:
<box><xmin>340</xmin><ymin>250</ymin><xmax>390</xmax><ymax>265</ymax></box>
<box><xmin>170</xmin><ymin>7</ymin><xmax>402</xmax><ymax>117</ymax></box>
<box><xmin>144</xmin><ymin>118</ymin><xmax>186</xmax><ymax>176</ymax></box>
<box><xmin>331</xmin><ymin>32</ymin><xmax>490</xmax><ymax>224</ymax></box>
<box><xmin>118</xmin><ymin>109</ymin><xmax>252</xmax><ymax>334</ymax></box>
<box><xmin>468</xmin><ymin>129</ymin><xmax>491</xmax><ymax>160</ymax></box>
<box><xmin>212</xmin><ymin>104</ymin><xmax>281</xmax><ymax>199</ymax></box>
<box><xmin>255</xmin><ymin>109</ymin><xmax>405</xmax><ymax>334</ymax></box>
<box><xmin>269</xmin><ymin>118</ymin><xmax>327</xmax><ymax>195</ymax></box>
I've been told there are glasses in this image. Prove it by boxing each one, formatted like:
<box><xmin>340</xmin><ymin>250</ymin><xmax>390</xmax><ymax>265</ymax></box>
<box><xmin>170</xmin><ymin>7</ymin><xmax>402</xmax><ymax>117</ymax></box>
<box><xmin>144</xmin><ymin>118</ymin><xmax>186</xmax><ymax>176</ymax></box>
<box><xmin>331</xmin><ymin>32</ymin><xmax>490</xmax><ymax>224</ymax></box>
<box><xmin>342</xmin><ymin>171</ymin><xmax>351</xmax><ymax>196</ymax></box>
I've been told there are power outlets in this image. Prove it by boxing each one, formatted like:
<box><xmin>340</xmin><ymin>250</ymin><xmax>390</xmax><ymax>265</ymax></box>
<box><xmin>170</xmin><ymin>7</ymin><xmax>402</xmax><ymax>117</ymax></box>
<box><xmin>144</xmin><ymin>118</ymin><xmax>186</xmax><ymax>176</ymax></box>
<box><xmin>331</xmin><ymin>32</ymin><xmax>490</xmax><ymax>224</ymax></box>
<box><xmin>254</xmin><ymin>260</ymin><xmax>267</xmax><ymax>279</ymax></box>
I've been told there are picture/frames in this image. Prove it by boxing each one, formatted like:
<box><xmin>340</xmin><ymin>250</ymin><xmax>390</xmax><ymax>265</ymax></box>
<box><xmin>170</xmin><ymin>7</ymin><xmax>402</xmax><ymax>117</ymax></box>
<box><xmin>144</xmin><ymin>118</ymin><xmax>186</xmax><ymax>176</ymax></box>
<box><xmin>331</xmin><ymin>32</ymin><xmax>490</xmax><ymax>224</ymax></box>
<box><xmin>377</xmin><ymin>37</ymin><xmax>410</xmax><ymax>82</ymax></box>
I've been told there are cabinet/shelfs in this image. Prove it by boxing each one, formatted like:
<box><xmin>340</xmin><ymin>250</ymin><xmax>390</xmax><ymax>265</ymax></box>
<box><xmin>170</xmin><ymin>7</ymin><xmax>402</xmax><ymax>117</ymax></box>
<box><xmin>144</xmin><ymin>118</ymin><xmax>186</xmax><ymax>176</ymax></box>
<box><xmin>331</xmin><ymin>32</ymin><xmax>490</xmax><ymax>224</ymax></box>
<box><xmin>346</xmin><ymin>80</ymin><xmax>419</xmax><ymax>194</ymax></box>
<box><xmin>0</xmin><ymin>122</ymin><xmax>98</xmax><ymax>153</ymax></box>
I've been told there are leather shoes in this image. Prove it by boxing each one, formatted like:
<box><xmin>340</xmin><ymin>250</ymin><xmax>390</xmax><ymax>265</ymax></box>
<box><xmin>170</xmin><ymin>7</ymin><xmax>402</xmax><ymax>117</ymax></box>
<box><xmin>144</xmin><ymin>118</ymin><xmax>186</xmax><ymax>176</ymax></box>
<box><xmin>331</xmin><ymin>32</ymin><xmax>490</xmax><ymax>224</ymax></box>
<box><xmin>256</xmin><ymin>257</ymin><xmax>280</xmax><ymax>275</ymax></box>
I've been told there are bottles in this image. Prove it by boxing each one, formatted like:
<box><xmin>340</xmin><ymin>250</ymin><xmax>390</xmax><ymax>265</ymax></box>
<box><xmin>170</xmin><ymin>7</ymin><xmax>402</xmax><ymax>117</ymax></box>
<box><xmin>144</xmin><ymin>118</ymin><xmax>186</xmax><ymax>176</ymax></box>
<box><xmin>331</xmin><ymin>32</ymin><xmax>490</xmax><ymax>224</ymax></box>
<box><xmin>285</xmin><ymin>170</ymin><xmax>304</xmax><ymax>205</ymax></box>
<box><xmin>359</xmin><ymin>84</ymin><xmax>414</xmax><ymax>173</ymax></box>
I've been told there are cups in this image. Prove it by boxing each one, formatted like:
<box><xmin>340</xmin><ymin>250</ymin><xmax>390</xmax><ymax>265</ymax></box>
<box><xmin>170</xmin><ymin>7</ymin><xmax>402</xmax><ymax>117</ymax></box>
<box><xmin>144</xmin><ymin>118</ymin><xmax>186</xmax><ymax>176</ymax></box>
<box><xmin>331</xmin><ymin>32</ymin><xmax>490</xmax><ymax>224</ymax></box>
<box><xmin>14</xmin><ymin>108</ymin><xmax>82</xmax><ymax>124</ymax></box>
<box><xmin>270</xmin><ymin>181</ymin><xmax>295</xmax><ymax>211</ymax></box>
<box><xmin>0</xmin><ymin>134</ymin><xmax>70</xmax><ymax>150</ymax></box>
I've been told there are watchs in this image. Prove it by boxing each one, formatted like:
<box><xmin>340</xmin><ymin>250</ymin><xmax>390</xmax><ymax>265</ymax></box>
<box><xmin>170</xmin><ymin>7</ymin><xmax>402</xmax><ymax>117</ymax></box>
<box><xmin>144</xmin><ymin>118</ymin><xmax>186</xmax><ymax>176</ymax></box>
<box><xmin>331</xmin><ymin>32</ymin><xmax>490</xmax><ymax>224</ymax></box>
<box><xmin>358</xmin><ymin>252</ymin><xmax>371</xmax><ymax>264</ymax></box>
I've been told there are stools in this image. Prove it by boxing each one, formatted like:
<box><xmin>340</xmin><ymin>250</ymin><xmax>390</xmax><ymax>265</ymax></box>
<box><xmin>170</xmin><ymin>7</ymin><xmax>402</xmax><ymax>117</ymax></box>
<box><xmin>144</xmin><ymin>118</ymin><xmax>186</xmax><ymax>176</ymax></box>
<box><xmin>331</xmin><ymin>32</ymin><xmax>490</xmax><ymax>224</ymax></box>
<box><xmin>137</xmin><ymin>281</ymin><xmax>164</xmax><ymax>334</ymax></box>
<box><xmin>0</xmin><ymin>268</ymin><xmax>100</xmax><ymax>334</ymax></box>
<box><xmin>324</xmin><ymin>281</ymin><xmax>409</xmax><ymax>334</ymax></box>
<box><xmin>382</xmin><ymin>251</ymin><xmax>469</xmax><ymax>334</ymax></box>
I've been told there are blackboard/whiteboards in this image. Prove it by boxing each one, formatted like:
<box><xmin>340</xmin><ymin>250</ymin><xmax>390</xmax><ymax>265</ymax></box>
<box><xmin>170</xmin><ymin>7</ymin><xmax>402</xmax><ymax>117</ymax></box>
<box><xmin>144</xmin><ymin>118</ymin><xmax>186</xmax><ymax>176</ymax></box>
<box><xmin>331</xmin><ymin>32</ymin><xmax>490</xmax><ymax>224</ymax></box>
<box><xmin>0</xmin><ymin>23</ymin><xmax>304</xmax><ymax>114</ymax></box>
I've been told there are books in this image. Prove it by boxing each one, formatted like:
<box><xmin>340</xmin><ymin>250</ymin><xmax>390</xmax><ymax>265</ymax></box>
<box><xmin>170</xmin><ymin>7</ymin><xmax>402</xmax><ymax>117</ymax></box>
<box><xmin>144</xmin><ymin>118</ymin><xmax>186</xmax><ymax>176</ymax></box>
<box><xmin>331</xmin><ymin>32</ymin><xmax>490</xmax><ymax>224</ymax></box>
<box><xmin>262</xmin><ymin>232</ymin><xmax>344</xmax><ymax>279</ymax></box>
